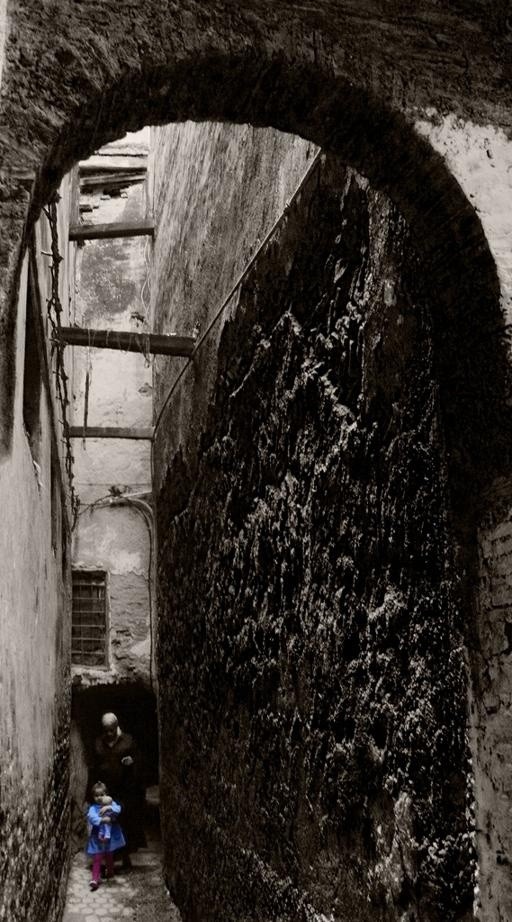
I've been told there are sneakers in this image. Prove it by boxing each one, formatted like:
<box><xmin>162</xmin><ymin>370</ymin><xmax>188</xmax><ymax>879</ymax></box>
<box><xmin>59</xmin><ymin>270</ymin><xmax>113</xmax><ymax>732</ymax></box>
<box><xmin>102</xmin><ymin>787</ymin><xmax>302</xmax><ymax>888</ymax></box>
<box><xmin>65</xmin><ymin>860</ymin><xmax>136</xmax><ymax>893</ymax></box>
<box><xmin>90</xmin><ymin>880</ymin><xmax>99</xmax><ymax>888</ymax></box>
<box><xmin>117</xmin><ymin>857</ymin><xmax>132</xmax><ymax>874</ymax></box>
<box><xmin>107</xmin><ymin>877</ymin><xmax>117</xmax><ymax>884</ymax></box>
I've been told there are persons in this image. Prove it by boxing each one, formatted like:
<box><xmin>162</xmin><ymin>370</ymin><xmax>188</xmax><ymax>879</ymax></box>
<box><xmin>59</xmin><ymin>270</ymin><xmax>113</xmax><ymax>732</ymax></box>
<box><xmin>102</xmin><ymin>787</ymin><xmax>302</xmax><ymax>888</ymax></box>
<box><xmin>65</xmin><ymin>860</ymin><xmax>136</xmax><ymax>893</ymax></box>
<box><xmin>79</xmin><ymin>712</ymin><xmax>137</xmax><ymax>888</ymax></box>
